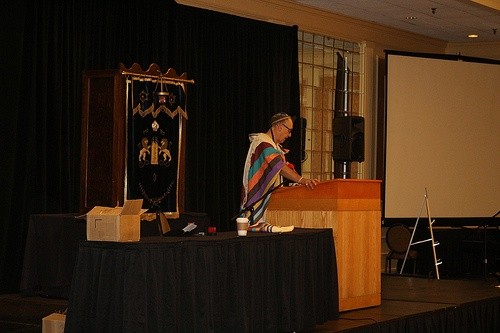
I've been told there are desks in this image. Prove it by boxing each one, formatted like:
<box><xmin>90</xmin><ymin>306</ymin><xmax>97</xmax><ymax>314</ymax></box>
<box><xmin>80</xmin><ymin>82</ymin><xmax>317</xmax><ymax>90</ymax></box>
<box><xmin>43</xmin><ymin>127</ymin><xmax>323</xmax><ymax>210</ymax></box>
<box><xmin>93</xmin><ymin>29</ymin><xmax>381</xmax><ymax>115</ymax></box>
<box><xmin>66</xmin><ymin>227</ymin><xmax>339</xmax><ymax>333</ymax></box>
<box><xmin>20</xmin><ymin>212</ymin><xmax>207</xmax><ymax>298</ymax></box>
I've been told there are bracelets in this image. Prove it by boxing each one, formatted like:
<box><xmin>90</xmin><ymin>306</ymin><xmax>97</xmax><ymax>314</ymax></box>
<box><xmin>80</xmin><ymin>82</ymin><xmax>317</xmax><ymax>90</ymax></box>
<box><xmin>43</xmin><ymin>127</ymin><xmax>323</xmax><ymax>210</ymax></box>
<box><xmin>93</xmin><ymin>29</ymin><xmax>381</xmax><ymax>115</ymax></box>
<box><xmin>298</xmin><ymin>177</ymin><xmax>303</xmax><ymax>184</ymax></box>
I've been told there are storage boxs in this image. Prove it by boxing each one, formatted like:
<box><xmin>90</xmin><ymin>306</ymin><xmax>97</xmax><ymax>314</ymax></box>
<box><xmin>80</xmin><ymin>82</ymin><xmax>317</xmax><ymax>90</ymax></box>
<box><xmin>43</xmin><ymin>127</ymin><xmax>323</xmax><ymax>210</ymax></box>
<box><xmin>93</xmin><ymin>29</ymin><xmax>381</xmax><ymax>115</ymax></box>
<box><xmin>75</xmin><ymin>199</ymin><xmax>149</xmax><ymax>243</ymax></box>
<box><xmin>41</xmin><ymin>313</ymin><xmax>66</xmax><ymax>333</ymax></box>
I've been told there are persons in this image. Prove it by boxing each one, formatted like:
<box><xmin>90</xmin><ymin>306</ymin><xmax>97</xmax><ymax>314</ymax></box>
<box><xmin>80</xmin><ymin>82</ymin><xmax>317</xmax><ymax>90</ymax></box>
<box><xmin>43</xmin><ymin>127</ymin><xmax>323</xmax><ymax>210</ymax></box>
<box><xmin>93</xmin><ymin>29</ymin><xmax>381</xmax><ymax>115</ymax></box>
<box><xmin>239</xmin><ymin>113</ymin><xmax>318</xmax><ymax>227</ymax></box>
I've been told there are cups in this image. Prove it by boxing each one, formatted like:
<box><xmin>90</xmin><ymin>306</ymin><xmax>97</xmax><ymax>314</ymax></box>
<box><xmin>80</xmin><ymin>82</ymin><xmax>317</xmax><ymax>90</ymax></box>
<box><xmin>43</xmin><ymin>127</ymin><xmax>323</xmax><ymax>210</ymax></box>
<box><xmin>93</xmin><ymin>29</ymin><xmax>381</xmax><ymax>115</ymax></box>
<box><xmin>236</xmin><ymin>217</ymin><xmax>249</xmax><ymax>236</ymax></box>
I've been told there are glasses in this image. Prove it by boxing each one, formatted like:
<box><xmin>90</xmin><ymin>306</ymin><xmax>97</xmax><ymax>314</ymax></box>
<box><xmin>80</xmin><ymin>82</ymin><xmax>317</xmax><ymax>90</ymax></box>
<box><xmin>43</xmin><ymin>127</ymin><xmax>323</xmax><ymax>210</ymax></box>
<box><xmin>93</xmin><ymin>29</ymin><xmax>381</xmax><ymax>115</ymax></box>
<box><xmin>277</xmin><ymin>124</ymin><xmax>293</xmax><ymax>134</ymax></box>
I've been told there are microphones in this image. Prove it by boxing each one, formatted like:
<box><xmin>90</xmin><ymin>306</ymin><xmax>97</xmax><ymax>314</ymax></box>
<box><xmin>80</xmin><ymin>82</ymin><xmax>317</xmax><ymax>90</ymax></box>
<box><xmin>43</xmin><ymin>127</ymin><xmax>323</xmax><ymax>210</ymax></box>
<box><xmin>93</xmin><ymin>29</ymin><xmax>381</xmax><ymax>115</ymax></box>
<box><xmin>288</xmin><ymin>163</ymin><xmax>295</xmax><ymax>171</ymax></box>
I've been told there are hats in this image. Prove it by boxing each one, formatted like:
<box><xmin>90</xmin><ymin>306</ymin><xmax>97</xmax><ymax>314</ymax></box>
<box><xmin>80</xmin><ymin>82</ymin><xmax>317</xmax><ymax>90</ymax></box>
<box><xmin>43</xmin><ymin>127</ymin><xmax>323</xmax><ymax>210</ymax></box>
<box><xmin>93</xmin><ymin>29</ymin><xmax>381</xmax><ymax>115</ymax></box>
<box><xmin>271</xmin><ymin>113</ymin><xmax>291</xmax><ymax>125</ymax></box>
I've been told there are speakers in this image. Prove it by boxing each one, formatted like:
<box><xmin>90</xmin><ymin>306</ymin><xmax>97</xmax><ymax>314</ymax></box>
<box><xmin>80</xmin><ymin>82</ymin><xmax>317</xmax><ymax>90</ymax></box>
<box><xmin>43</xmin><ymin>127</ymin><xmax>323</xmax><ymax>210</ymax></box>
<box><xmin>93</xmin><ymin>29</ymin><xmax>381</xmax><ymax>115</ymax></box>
<box><xmin>332</xmin><ymin>116</ymin><xmax>365</xmax><ymax>163</ymax></box>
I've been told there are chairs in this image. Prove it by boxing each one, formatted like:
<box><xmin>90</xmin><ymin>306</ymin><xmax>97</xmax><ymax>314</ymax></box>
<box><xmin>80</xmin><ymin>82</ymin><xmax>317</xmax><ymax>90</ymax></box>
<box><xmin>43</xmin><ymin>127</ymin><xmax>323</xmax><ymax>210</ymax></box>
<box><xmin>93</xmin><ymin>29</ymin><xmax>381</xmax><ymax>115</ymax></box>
<box><xmin>384</xmin><ymin>226</ymin><xmax>417</xmax><ymax>274</ymax></box>
